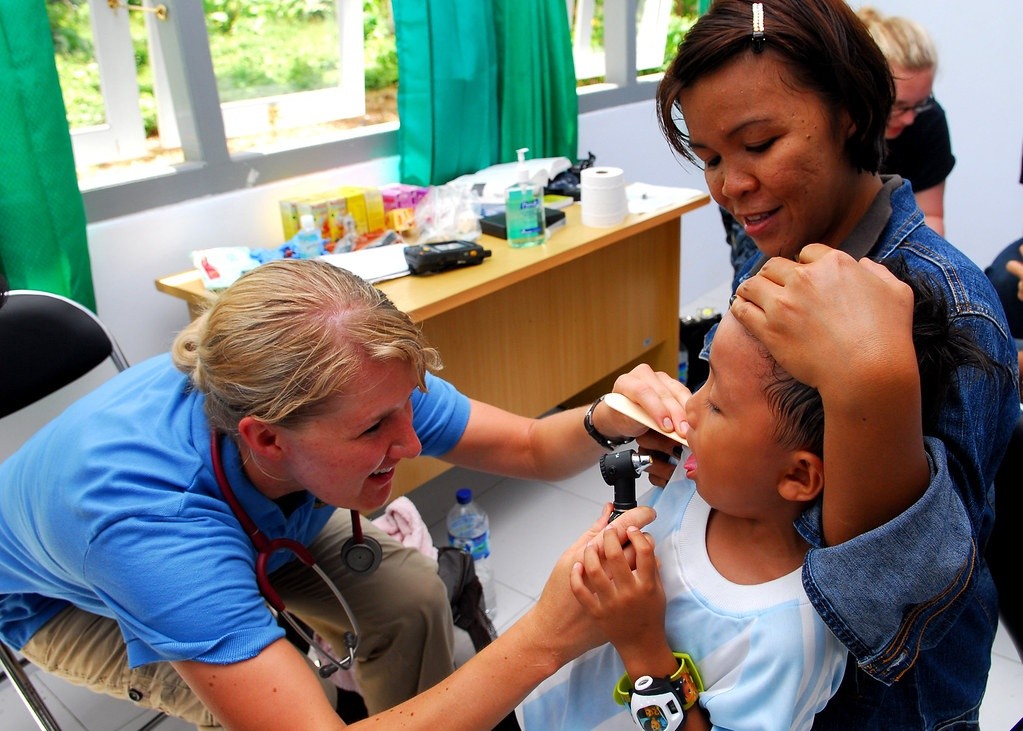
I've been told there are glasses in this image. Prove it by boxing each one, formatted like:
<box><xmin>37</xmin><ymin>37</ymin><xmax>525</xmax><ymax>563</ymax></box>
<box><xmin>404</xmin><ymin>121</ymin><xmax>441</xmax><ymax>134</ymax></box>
<box><xmin>890</xmin><ymin>91</ymin><xmax>935</xmax><ymax>121</ymax></box>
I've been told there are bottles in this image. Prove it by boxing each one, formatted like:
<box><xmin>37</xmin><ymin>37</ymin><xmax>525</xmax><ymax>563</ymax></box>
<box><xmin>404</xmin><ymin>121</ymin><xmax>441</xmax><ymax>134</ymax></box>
<box><xmin>445</xmin><ymin>488</ymin><xmax>498</xmax><ymax>621</ymax></box>
<box><xmin>296</xmin><ymin>214</ymin><xmax>323</xmax><ymax>259</ymax></box>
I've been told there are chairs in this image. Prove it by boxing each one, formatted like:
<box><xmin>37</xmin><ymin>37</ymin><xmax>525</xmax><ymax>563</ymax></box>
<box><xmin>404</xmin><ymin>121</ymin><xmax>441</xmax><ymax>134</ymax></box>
<box><xmin>0</xmin><ymin>288</ymin><xmax>167</xmax><ymax>731</ymax></box>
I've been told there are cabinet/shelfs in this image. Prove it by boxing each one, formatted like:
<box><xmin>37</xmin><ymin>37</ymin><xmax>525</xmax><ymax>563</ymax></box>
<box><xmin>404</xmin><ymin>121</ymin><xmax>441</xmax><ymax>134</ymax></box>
<box><xmin>155</xmin><ymin>183</ymin><xmax>711</xmax><ymax>519</ymax></box>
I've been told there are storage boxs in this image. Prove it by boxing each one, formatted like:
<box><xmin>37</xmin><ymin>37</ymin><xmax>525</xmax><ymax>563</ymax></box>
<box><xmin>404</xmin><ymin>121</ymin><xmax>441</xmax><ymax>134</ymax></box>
<box><xmin>278</xmin><ymin>183</ymin><xmax>428</xmax><ymax>243</ymax></box>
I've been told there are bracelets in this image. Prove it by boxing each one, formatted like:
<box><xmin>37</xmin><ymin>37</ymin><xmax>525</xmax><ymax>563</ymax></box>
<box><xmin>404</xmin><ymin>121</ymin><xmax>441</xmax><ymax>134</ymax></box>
<box><xmin>583</xmin><ymin>394</ymin><xmax>636</xmax><ymax>452</ymax></box>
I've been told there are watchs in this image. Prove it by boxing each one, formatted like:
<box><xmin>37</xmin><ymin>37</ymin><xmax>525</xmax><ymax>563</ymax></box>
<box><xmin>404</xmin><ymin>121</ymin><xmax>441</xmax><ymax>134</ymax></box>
<box><xmin>613</xmin><ymin>653</ymin><xmax>703</xmax><ymax>731</ymax></box>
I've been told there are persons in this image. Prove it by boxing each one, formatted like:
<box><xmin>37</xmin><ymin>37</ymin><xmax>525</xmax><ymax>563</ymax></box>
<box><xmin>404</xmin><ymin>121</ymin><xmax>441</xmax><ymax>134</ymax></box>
<box><xmin>720</xmin><ymin>7</ymin><xmax>955</xmax><ymax>280</ymax></box>
<box><xmin>636</xmin><ymin>1</ymin><xmax>1021</xmax><ymax>731</ymax></box>
<box><xmin>0</xmin><ymin>260</ymin><xmax>692</xmax><ymax>731</ymax></box>
<box><xmin>984</xmin><ymin>238</ymin><xmax>1023</xmax><ymax>731</ymax></box>
<box><xmin>337</xmin><ymin>254</ymin><xmax>1009</xmax><ymax>731</ymax></box>
